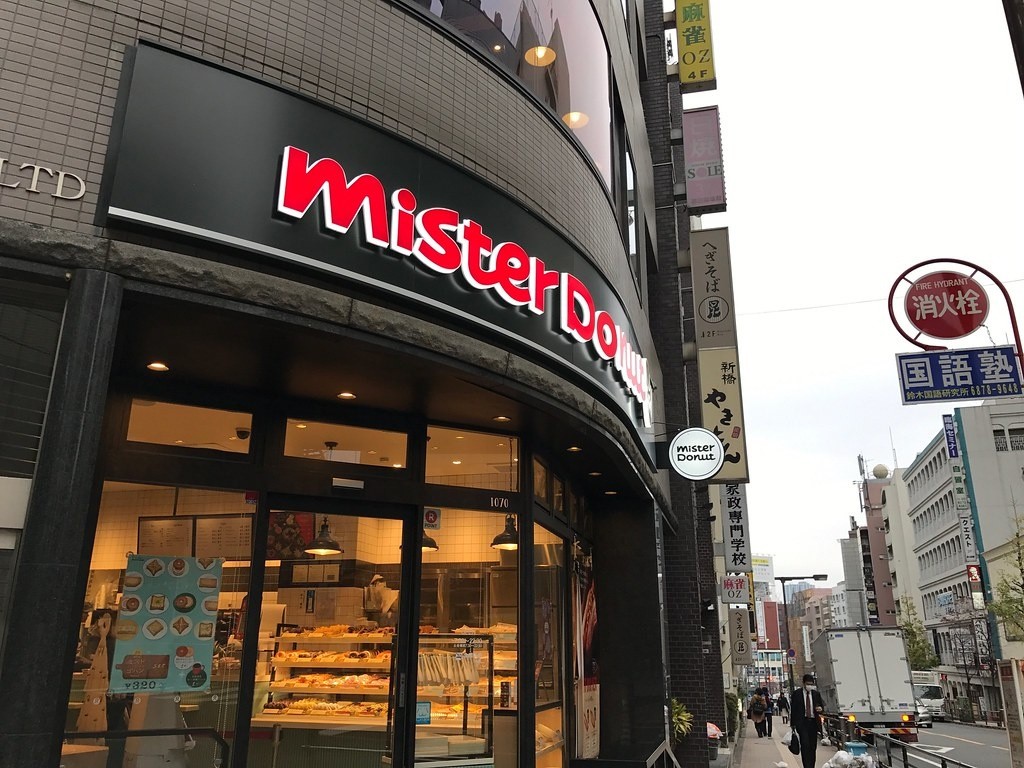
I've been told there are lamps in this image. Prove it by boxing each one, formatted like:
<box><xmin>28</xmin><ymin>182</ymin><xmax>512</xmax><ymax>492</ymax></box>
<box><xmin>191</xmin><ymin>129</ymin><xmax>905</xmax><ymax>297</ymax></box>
<box><xmin>524</xmin><ymin>44</ymin><xmax>557</xmax><ymax>68</ymax></box>
<box><xmin>561</xmin><ymin>111</ymin><xmax>590</xmax><ymax>130</ymax></box>
<box><xmin>490</xmin><ymin>438</ymin><xmax>519</xmax><ymax>551</ymax></box>
<box><xmin>303</xmin><ymin>442</ymin><xmax>343</xmax><ymax>554</ymax></box>
<box><xmin>398</xmin><ymin>436</ymin><xmax>439</xmax><ymax>552</ymax></box>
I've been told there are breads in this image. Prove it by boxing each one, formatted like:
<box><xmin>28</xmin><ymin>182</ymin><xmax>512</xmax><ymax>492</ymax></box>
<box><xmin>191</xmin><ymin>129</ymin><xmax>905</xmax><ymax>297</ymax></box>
<box><xmin>264</xmin><ymin>624</ymin><xmax>395</xmax><ymax>715</ymax></box>
<box><xmin>419</xmin><ymin>623</ymin><xmax>516</xmax><ymax>713</ymax></box>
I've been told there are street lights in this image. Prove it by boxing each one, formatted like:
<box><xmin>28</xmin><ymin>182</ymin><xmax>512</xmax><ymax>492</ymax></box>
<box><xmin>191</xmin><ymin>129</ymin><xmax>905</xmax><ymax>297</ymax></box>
<box><xmin>774</xmin><ymin>574</ymin><xmax>828</xmax><ymax>695</ymax></box>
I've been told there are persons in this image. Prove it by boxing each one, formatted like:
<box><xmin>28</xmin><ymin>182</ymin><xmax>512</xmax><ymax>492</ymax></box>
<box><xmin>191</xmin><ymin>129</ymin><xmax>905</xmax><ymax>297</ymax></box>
<box><xmin>790</xmin><ymin>674</ymin><xmax>824</xmax><ymax>768</ymax></box>
<box><xmin>777</xmin><ymin>693</ymin><xmax>790</xmax><ymax>724</ymax></box>
<box><xmin>750</xmin><ymin>687</ymin><xmax>774</xmax><ymax>739</ymax></box>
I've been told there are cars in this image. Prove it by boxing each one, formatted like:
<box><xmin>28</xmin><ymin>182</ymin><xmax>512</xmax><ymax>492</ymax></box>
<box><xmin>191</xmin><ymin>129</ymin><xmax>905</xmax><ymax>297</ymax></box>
<box><xmin>914</xmin><ymin>697</ymin><xmax>932</xmax><ymax>728</ymax></box>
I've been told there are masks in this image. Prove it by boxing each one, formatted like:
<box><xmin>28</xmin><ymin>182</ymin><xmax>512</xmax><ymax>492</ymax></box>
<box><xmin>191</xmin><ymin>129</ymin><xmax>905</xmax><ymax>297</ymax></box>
<box><xmin>804</xmin><ymin>682</ymin><xmax>813</xmax><ymax>691</ymax></box>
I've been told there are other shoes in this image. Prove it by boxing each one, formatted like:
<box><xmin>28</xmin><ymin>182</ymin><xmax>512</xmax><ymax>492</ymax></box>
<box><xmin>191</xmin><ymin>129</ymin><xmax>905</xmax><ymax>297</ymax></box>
<box><xmin>768</xmin><ymin>736</ymin><xmax>772</xmax><ymax>740</ymax></box>
<box><xmin>765</xmin><ymin>732</ymin><xmax>768</xmax><ymax>736</ymax></box>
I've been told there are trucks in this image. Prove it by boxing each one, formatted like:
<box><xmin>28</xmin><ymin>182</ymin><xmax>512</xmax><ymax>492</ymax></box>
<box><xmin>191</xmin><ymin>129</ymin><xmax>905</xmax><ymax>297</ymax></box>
<box><xmin>911</xmin><ymin>671</ymin><xmax>950</xmax><ymax>722</ymax></box>
<box><xmin>811</xmin><ymin>627</ymin><xmax>918</xmax><ymax>748</ymax></box>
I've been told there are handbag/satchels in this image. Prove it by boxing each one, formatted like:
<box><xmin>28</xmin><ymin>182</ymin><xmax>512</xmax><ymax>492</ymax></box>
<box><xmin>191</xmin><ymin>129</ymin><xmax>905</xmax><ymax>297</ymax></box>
<box><xmin>781</xmin><ymin>728</ymin><xmax>802</xmax><ymax>755</ymax></box>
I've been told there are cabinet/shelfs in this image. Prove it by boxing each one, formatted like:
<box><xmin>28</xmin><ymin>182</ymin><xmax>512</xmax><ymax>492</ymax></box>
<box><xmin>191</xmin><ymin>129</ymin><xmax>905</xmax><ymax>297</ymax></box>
<box><xmin>420</xmin><ymin>573</ymin><xmax>484</xmax><ymax>630</ymax></box>
<box><xmin>249</xmin><ymin>622</ymin><xmax>494</xmax><ymax>768</ymax></box>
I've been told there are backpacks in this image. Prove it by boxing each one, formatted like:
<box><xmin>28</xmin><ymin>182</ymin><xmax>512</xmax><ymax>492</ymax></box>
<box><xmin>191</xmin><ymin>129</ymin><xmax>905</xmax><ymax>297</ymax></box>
<box><xmin>753</xmin><ymin>695</ymin><xmax>764</xmax><ymax>714</ymax></box>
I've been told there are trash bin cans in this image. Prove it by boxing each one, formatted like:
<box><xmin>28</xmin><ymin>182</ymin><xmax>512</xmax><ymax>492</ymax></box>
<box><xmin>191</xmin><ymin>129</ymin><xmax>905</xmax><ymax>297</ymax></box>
<box><xmin>844</xmin><ymin>742</ymin><xmax>868</xmax><ymax>756</ymax></box>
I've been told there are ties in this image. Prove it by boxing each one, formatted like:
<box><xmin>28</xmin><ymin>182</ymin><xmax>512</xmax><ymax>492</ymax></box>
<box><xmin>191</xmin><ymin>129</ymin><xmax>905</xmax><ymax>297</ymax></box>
<box><xmin>806</xmin><ymin>691</ymin><xmax>811</xmax><ymax>719</ymax></box>
